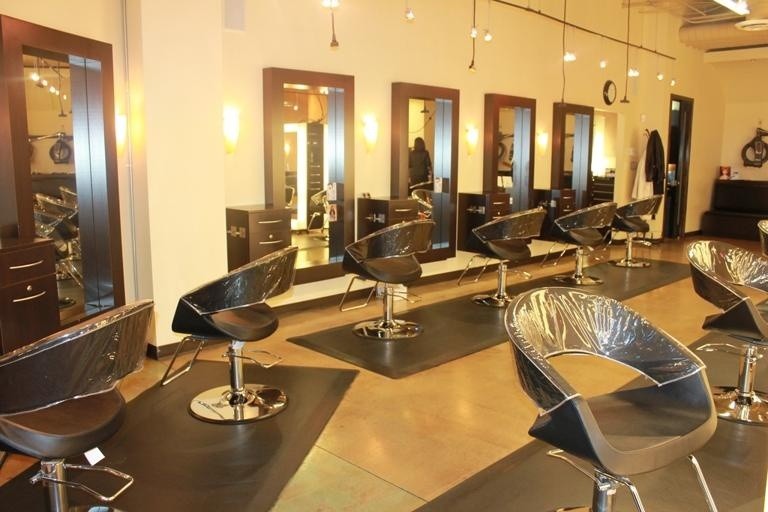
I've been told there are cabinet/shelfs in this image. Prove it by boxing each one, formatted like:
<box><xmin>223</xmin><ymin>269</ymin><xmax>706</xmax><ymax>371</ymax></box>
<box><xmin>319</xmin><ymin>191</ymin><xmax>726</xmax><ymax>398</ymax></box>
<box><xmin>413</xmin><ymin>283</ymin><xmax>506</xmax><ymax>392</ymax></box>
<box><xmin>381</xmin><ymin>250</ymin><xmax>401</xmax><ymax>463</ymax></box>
<box><xmin>432</xmin><ymin>192</ymin><xmax>450</xmax><ymax>243</ymax></box>
<box><xmin>551</xmin><ymin>188</ymin><xmax>576</xmax><ymax>218</ymax></box>
<box><xmin>458</xmin><ymin>192</ymin><xmax>510</xmax><ymax>253</ymax></box>
<box><xmin>0</xmin><ymin>237</ymin><xmax>61</xmax><ymax>355</ymax></box>
<box><xmin>328</xmin><ymin>182</ymin><xmax>344</xmax><ymax>261</ymax></box>
<box><xmin>357</xmin><ymin>197</ymin><xmax>418</xmax><ymax>241</ymax></box>
<box><xmin>226</xmin><ymin>204</ymin><xmax>292</xmax><ymax>274</ymax></box>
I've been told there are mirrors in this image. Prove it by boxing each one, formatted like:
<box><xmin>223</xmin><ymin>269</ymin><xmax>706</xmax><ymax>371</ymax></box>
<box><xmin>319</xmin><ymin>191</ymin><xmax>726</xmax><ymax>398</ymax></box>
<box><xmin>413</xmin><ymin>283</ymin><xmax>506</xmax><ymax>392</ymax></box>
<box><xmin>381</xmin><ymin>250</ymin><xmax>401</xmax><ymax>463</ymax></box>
<box><xmin>391</xmin><ymin>82</ymin><xmax>460</xmax><ymax>263</ymax></box>
<box><xmin>483</xmin><ymin>93</ymin><xmax>536</xmax><ymax>212</ymax></box>
<box><xmin>262</xmin><ymin>67</ymin><xmax>355</xmax><ymax>286</ymax></box>
<box><xmin>551</xmin><ymin>103</ymin><xmax>594</xmax><ymax>208</ymax></box>
<box><xmin>1</xmin><ymin>14</ymin><xmax>125</xmax><ymax>329</ymax></box>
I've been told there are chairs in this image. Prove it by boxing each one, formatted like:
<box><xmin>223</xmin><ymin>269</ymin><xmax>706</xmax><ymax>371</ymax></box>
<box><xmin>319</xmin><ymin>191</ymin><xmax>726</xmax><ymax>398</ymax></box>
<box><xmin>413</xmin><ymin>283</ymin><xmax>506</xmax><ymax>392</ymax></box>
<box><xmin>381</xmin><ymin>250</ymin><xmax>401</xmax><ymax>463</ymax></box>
<box><xmin>339</xmin><ymin>219</ymin><xmax>436</xmax><ymax>341</ymax></box>
<box><xmin>158</xmin><ymin>245</ymin><xmax>299</xmax><ymax>424</ymax></box>
<box><xmin>34</xmin><ymin>186</ymin><xmax>83</xmax><ymax>308</ymax></box>
<box><xmin>504</xmin><ymin>288</ymin><xmax>718</xmax><ymax>512</ymax></box>
<box><xmin>684</xmin><ymin>239</ymin><xmax>768</xmax><ymax>424</ymax></box>
<box><xmin>0</xmin><ymin>298</ymin><xmax>154</xmax><ymax>512</ymax></box>
<box><xmin>594</xmin><ymin>195</ymin><xmax>662</xmax><ymax>268</ymax></box>
<box><xmin>285</xmin><ymin>186</ymin><xmax>329</xmax><ymax>241</ymax></box>
<box><xmin>457</xmin><ymin>209</ymin><xmax>548</xmax><ymax>307</ymax></box>
<box><xmin>411</xmin><ymin>189</ymin><xmax>432</xmax><ymax>219</ymax></box>
<box><xmin>540</xmin><ymin>202</ymin><xmax>617</xmax><ymax>287</ymax></box>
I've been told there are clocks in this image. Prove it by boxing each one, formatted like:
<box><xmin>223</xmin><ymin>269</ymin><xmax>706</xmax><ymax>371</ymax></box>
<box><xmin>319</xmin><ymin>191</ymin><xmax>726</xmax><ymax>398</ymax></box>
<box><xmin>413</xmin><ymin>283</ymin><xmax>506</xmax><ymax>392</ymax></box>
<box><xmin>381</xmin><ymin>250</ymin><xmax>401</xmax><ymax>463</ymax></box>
<box><xmin>603</xmin><ymin>81</ymin><xmax>616</xmax><ymax>106</ymax></box>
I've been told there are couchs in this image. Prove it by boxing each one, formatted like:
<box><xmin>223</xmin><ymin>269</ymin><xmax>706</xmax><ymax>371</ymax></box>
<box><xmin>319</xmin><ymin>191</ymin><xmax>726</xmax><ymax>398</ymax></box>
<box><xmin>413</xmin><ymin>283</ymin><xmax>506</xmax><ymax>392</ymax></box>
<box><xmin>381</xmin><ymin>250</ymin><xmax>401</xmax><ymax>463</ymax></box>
<box><xmin>701</xmin><ymin>179</ymin><xmax>768</xmax><ymax>240</ymax></box>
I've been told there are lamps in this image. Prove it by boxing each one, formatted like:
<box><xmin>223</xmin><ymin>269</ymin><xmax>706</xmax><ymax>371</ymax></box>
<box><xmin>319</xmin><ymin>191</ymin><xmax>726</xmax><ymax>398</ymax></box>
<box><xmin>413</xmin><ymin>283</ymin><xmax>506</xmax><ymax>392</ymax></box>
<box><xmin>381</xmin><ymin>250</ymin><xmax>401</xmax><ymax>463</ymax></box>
<box><xmin>620</xmin><ymin>2</ymin><xmax>633</xmax><ymax>104</ymax></box>
<box><xmin>468</xmin><ymin>1</ymin><xmax>478</xmax><ymax>73</ymax></box>
<box><xmin>656</xmin><ymin>56</ymin><xmax>664</xmax><ymax>81</ymax></box>
<box><xmin>405</xmin><ymin>2</ymin><xmax>415</xmax><ymax>23</ymax></box>
<box><xmin>30</xmin><ymin>73</ymin><xmax>66</xmax><ymax>99</ymax></box>
<box><xmin>557</xmin><ymin>1</ymin><xmax>568</xmax><ymax>109</ymax></box>
<box><xmin>599</xmin><ymin>35</ymin><xmax>606</xmax><ymax>69</ymax></box>
<box><xmin>628</xmin><ymin>46</ymin><xmax>639</xmax><ymax>79</ymax></box>
<box><xmin>671</xmin><ymin>61</ymin><xmax>674</xmax><ymax>86</ymax></box>
<box><xmin>328</xmin><ymin>2</ymin><xmax>340</xmax><ymax>48</ymax></box>
<box><xmin>562</xmin><ymin>26</ymin><xmax>577</xmax><ymax>63</ymax></box>
<box><xmin>483</xmin><ymin>1</ymin><xmax>494</xmax><ymax>42</ymax></box>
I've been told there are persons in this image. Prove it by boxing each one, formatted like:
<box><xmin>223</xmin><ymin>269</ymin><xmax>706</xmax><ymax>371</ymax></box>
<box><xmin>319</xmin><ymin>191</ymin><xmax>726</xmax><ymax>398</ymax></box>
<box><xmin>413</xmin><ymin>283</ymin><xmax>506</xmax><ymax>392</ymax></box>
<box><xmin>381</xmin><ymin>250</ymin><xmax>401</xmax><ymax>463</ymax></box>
<box><xmin>409</xmin><ymin>137</ymin><xmax>433</xmax><ymax>190</ymax></box>
